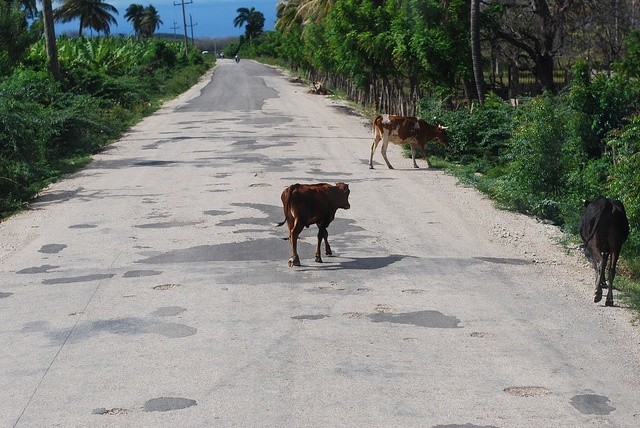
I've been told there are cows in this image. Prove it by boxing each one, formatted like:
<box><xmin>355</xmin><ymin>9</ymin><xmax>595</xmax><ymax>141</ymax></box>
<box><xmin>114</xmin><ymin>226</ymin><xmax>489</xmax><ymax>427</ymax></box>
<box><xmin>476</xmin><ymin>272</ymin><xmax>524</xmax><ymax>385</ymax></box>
<box><xmin>580</xmin><ymin>196</ymin><xmax>628</xmax><ymax>307</ymax></box>
<box><xmin>274</xmin><ymin>182</ymin><xmax>350</xmax><ymax>268</ymax></box>
<box><xmin>368</xmin><ymin>114</ymin><xmax>450</xmax><ymax>169</ymax></box>
